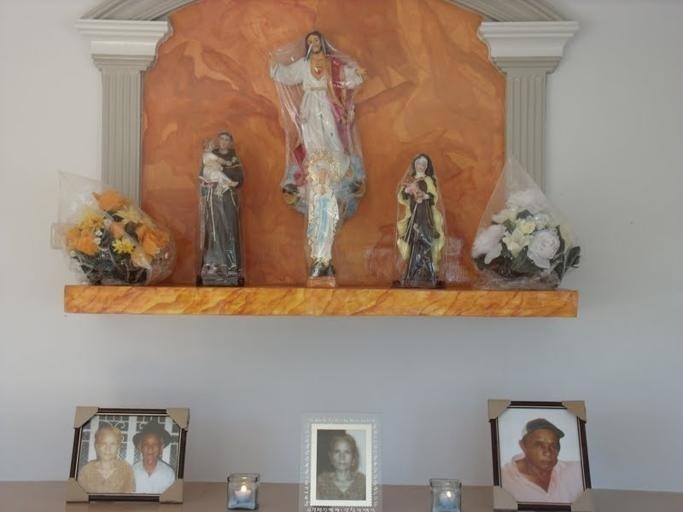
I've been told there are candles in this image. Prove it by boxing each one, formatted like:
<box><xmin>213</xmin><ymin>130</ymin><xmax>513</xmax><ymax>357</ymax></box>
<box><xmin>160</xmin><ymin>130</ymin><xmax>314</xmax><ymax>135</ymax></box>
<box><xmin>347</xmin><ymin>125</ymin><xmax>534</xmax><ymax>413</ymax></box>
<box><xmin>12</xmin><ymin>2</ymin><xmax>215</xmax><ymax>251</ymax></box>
<box><xmin>227</xmin><ymin>473</ymin><xmax>259</xmax><ymax>509</ymax></box>
<box><xmin>430</xmin><ymin>479</ymin><xmax>463</xmax><ymax>512</ymax></box>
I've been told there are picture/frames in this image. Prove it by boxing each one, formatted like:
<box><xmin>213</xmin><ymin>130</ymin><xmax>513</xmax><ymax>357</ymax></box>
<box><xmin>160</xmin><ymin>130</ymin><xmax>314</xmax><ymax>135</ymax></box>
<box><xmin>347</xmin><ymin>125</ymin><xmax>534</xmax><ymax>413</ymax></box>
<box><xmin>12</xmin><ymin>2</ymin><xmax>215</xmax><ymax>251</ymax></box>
<box><xmin>488</xmin><ymin>401</ymin><xmax>591</xmax><ymax>512</ymax></box>
<box><xmin>299</xmin><ymin>411</ymin><xmax>382</xmax><ymax>512</ymax></box>
<box><xmin>64</xmin><ymin>406</ymin><xmax>189</xmax><ymax>502</ymax></box>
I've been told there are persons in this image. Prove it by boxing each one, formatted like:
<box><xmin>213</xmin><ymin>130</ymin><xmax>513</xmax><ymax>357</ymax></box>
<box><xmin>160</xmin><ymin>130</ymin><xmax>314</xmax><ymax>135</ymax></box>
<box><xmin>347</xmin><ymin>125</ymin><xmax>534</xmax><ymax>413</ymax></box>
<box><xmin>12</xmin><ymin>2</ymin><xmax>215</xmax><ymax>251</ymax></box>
<box><xmin>196</xmin><ymin>131</ymin><xmax>242</xmax><ymax>287</ymax></box>
<box><xmin>500</xmin><ymin>418</ymin><xmax>584</xmax><ymax>504</ymax></box>
<box><xmin>394</xmin><ymin>152</ymin><xmax>448</xmax><ymax>289</ymax></box>
<box><xmin>77</xmin><ymin>423</ymin><xmax>136</xmax><ymax>494</ymax></box>
<box><xmin>270</xmin><ymin>31</ymin><xmax>368</xmax><ymax>276</ymax></box>
<box><xmin>130</xmin><ymin>423</ymin><xmax>176</xmax><ymax>494</ymax></box>
<box><xmin>317</xmin><ymin>433</ymin><xmax>366</xmax><ymax>500</ymax></box>
<box><xmin>202</xmin><ymin>135</ymin><xmax>239</xmax><ymax>196</ymax></box>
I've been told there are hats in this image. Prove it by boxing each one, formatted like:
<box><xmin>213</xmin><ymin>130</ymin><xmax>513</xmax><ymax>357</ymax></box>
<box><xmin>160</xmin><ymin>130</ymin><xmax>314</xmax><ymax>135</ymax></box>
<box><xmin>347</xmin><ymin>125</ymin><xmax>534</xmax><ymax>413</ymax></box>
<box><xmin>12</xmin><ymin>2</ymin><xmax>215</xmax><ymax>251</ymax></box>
<box><xmin>527</xmin><ymin>419</ymin><xmax>565</xmax><ymax>438</ymax></box>
<box><xmin>133</xmin><ymin>422</ymin><xmax>172</xmax><ymax>448</ymax></box>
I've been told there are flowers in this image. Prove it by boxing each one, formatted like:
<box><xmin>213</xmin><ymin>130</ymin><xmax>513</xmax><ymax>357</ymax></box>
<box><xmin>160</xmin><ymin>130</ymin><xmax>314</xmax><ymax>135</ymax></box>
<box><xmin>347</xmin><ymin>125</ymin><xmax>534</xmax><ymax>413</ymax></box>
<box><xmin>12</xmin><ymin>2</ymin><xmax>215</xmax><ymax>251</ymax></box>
<box><xmin>61</xmin><ymin>188</ymin><xmax>172</xmax><ymax>271</ymax></box>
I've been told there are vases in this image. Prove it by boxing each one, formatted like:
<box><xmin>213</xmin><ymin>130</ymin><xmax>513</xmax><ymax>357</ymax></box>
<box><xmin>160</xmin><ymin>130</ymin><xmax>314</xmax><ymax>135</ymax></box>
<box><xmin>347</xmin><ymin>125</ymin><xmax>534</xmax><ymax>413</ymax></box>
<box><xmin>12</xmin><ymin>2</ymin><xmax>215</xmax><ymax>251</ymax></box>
<box><xmin>498</xmin><ymin>264</ymin><xmax>547</xmax><ymax>288</ymax></box>
<box><xmin>79</xmin><ymin>257</ymin><xmax>148</xmax><ymax>283</ymax></box>
<box><xmin>471</xmin><ymin>186</ymin><xmax>581</xmax><ymax>282</ymax></box>
